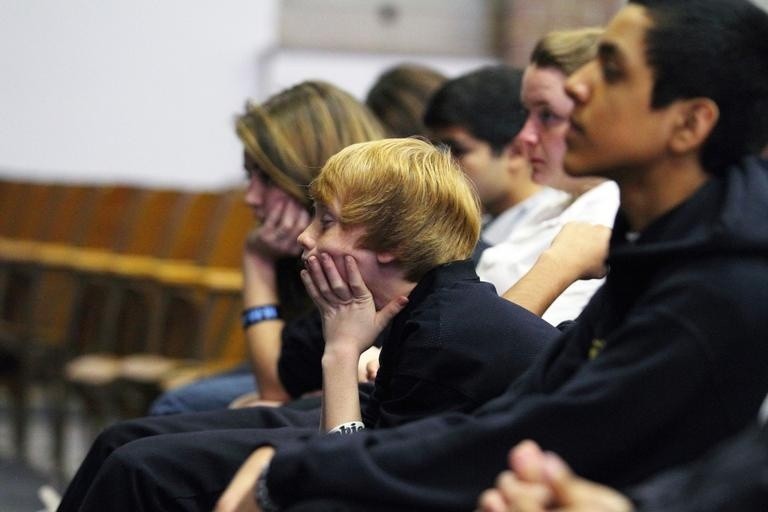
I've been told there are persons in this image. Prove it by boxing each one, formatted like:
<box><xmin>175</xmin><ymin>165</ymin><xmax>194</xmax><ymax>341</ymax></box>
<box><xmin>217</xmin><ymin>0</ymin><xmax>767</xmax><ymax>510</ymax></box>
<box><xmin>54</xmin><ymin>82</ymin><xmax>562</xmax><ymax>511</ymax></box>
